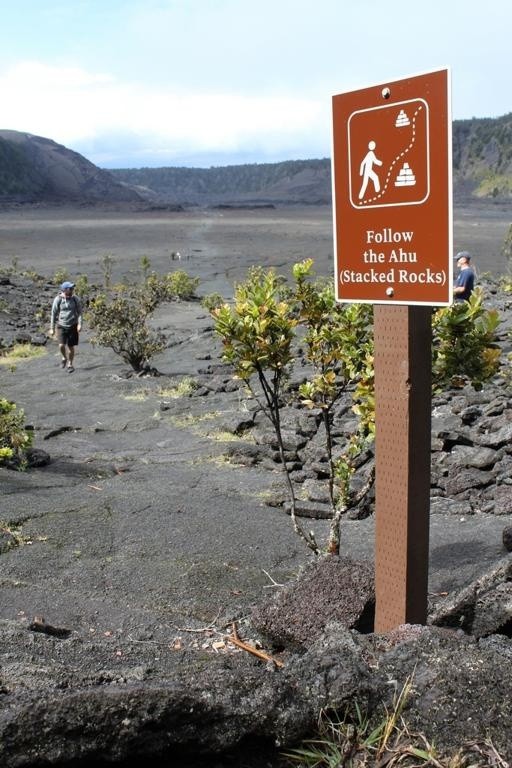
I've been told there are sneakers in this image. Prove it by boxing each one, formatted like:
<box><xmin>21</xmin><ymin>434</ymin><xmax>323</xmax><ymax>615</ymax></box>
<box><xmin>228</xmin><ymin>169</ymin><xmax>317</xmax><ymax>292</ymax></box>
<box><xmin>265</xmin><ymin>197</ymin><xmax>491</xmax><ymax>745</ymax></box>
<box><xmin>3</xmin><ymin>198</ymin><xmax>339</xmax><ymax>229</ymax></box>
<box><xmin>62</xmin><ymin>359</ymin><xmax>73</xmax><ymax>373</ymax></box>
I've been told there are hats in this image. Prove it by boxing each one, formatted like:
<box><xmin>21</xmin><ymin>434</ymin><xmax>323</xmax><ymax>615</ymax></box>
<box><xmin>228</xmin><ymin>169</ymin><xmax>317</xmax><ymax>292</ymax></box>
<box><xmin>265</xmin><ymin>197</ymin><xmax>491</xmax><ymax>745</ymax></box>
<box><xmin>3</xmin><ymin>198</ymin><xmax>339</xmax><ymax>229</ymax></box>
<box><xmin>453</xmin><ymin>252</ymin><xmax>471</xmax><ymax>260</ymax></box>
<box><xmin>62</xmin><ymin>282</ymin><xmax>76</xmax><ymax>288</ymax></box>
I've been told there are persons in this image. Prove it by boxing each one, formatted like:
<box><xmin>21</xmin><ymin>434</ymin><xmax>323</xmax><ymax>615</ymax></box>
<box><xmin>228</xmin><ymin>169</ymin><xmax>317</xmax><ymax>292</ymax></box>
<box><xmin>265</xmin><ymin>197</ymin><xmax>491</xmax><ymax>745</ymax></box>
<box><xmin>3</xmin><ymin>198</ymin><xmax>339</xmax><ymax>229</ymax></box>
<box><xmin>453</xmin><ymin>250</ymin><xmax>474</xmax><ymax>304</ymax></box>
<box><xmin>49</xmin><ymin>281</ymin><xmax>83</xmax><ymax>374</ymax></box>
